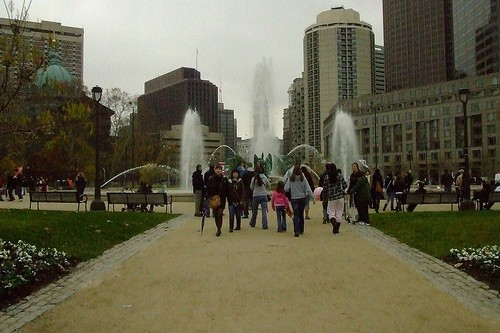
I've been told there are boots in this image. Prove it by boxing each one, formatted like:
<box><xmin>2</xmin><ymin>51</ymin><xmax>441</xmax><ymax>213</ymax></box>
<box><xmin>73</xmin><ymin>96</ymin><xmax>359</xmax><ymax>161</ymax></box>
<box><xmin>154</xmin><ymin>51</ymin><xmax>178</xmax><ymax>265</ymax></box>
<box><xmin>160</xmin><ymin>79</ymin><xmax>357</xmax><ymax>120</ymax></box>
<box><xmin>391</xmin><ymin>204</ymin><xmax>395</xmax><ymax>210</ymax></box>
<box><xmin>383</xmin><ymin>204</ymin><xmax>387</xmax><ymax>211</ymax></box>
<box><xmin>330</xmin><ymin>217</ymin><xmax>340</xmax><ymax>234</ymax></box>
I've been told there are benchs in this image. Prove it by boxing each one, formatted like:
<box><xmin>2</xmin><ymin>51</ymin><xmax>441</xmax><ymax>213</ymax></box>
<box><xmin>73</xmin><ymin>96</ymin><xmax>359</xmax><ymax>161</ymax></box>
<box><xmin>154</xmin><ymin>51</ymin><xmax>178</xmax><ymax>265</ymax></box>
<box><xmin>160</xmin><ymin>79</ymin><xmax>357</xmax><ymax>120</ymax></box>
<box><xmin>26</xmin><ymin>190</ymin><xmax>88</xmax><ymax>211</ymax></box>
<box><xmin>103</xmin><ymin>189</ymin><xmax>172</xmax><ymax>214</ymax></box>
<box><xmin>395</xmin><ymin>189</ymin><xmax>460</xmax><ymax>211</ymax></box>
<box><xmin>478</xmin><ymin>191</ymin><xmax>500</xmax><ymax>210</ymax></box>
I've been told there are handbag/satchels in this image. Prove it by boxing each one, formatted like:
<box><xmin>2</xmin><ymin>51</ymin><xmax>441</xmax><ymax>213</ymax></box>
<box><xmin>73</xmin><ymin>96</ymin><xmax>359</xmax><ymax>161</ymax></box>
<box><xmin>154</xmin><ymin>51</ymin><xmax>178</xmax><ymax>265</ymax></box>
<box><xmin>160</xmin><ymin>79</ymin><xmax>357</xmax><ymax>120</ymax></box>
<box><xmin>376</xmin><ymin>181</ymin><xmax>382</xmax><ymax>193</ymax></box>
<box><xmin>267</xmin><ymin>194</ymin><xmax>271</xmax><ymax>202</ymax></box>
<box><xmin>208</xmin><ymin>194</ymin><xmax>221</xmax><ymax>209</ymax></box>
<box><xmin>382</xmin><ymin>188</ymin><xmax>386</xmax><ymax>194</ymax></box>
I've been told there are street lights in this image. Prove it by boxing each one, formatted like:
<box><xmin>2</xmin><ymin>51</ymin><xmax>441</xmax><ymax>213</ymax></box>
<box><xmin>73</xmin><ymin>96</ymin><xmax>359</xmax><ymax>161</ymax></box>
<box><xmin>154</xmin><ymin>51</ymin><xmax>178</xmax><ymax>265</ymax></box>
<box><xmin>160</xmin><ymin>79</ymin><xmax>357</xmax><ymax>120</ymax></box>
<box><xmin>129</xmin><ymin>101</ymin><xmax>134</xmax><ymax>190</ymax></box>
<box><xmin>458</xmin><ymin>89</ymin><xmax>475</xmax><ymax>212</ymax></box>
<box><xmin>90</xmin><ymin>85</ymin><xmax>105</xmax><ymax>210</ymax></box>
<box><xmin>370</xmin><ymin>98</ymin><xmax>378</xmax><ymax>170</ymax></box>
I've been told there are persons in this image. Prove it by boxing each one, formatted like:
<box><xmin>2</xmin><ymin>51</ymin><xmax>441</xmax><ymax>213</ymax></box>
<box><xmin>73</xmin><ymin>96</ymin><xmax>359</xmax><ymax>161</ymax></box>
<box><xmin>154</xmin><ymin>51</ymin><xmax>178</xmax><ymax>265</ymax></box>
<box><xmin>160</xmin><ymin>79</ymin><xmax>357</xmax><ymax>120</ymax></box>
<box><xmin>271</xmin><ymin>181</ymin><xmax>290</xmax><ymax>232</ymax></box>
<box><xmin>284</xmin><ymin>166</ymin><xmax>316</xmax><ymax>237</ymax></box>
<box><xmin>0</xmin><ymin>168</ymin><xmax>87</xmax><ymax>203</ymax></box>
<box><xmin>441</xmin><ymin>169</ymin><xmax>500</xmax><ymax>210</ymax></box>
<box><xmin>322</xmin><ymin>163</ymin><xmax>348</xmax><ymax>234</ymax></box>
<box><xmin>319</xmin><ymin>161</ymin><xmax>427</xmax><ymax>224</ymax></box>
<box><xmin>283</xmin><ymin>164</ymin><xmax>315</xmax><ymax>220</ymax></box>
<box><xmin>249</xmin><ymin>166</ymin><xmax>271</xmax><ymax>230</ymax></box>
<box><xmin>347</xmin><ymin>170</ymin><xmax>371</xmax><ymax>225</ymax></box>
<box><xmin>192</xmin><ymin>161</ymin><xmax>264</xmax><ymax>218</ymax></box>
<box><xmin>137</xmin><ymin>181</ymin><xmax>154</xmax><ymax>212</ymax></box>
<box><xmin>204</xmin><ymin>165</ymin><xmax>227</xmax><ymax>236</ymax></box>
<box><xmin>226</xmin><ymin>168</ymin><xmax>244</xmax><ymax>233</ymax></box>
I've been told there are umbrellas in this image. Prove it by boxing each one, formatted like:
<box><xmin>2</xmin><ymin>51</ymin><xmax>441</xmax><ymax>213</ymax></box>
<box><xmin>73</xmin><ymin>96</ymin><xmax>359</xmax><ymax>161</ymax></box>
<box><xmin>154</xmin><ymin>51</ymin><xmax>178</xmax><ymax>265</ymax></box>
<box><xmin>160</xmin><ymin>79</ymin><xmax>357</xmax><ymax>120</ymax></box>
<box><xmin>201</xmin><ymin>200</ymin><xmax>208</xmax><ymax>235</ymax></box>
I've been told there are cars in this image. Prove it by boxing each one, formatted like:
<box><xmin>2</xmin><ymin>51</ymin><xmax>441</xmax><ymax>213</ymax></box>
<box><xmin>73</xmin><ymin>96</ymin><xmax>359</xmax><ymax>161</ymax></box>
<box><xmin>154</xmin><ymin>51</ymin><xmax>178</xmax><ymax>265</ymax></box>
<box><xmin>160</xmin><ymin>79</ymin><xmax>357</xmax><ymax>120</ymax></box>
<box><xmin>416</xmin><ymin>177</ymin><xmax>438</xmax><ymax>186</ymax></box>
<box><xmin>268</xmin><ymin>176</ymin><xmax>284</xmax><ymax>191</ymax></box>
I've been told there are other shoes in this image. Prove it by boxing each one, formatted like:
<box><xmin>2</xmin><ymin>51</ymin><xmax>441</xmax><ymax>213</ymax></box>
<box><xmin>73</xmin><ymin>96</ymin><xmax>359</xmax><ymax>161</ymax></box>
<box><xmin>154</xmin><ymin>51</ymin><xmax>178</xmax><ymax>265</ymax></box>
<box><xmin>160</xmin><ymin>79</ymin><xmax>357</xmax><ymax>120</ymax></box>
<box><xmin>19</xmin><ymin>199</ymin><xmax>22</xmax><ymax>202</ymax></box>
<box><xmin>234</xmin><ymin>227</ymin><xmax>239</xmax><ymax>230</ymax></box>
<box><xmin>9</xmin><ymin>199</ymin><xmax>15</xmax><ymax>201</ymax></box>
<box><xmin>322</xmin><ymin>216</ymin><xmax>327</xmax><ymax>224</ymax></box>
<box><xmin>263</xmin><ymin>226</ymin><xmax>268</xmax><ymax>229</ymax></box>
<box><xmin>242</xmin><ymin>215</ymin><xmax>249</xmax><ymax>218</ymax></box>
<box><xmin>305</xmin><ymin>216</ymin><xmax>310</xmax><ymax>220</ymax></box>
<box><xmin>278</xmin><ymin>229</ymin><xmax>281</xmax><ymax>232</ymax></box>
<box><xmin>282</xmin><ymin>228</ymin><xmax>285</xmax><ymax>230</ymax></box>
<box><xmin>216</xmin><ymin>230</ymin><xmax>221</xmax><ymax>236</ymax></box>
<box><xmin>229</xmin><ymin>228</ymin><xmax>233</xmax><ymax>232</ymax></box>
<box><xmin>249</xmin><ymin>222</ymin><xmax>255</xmax><ymax>227</ymax></box>
<box><xmin>0</xmin><ymin>199</ymin><xmax>4</xmax><ymax>201</ymax></box>
<box><xmin>295</xmin><ymin>232</ymin><xmax>299</xmax><ymax>237</ymax></box>
